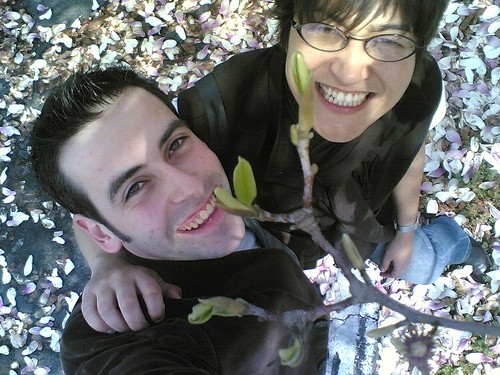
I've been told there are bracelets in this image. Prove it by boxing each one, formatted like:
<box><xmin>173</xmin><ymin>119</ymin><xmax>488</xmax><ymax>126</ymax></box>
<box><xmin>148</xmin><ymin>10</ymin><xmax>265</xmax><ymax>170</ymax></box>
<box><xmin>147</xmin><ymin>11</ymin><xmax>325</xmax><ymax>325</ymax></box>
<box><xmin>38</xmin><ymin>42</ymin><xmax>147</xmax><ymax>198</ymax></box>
<box><xmin>396</xmin><ymin>213</ymin><xmax>421</xmax><ymax>233</ymax></box>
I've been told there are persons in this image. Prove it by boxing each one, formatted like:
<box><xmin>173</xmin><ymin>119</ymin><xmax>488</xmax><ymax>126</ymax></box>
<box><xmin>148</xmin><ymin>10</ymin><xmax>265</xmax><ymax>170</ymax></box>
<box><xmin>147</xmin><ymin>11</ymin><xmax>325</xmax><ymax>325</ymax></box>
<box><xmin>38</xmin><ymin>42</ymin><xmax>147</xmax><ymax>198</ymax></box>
<box><xmin>33</xmin><ymin>67</ymin><xmax>329</xmax><ymax>374</ymax></box>
<box><xmin>73</xmin><ymin>0</ymin><xmax>490</xmax><ymax>332</ymax></box>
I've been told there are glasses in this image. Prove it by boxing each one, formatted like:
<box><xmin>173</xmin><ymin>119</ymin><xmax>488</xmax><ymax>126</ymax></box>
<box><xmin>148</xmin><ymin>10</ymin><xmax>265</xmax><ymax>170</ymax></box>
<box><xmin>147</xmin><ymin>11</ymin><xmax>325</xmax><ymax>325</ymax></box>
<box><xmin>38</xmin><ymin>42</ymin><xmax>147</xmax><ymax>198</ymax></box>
<box><xmin>289</xmin><ymin>18</ymin><xmax>424</xmax><ymax>62</ymax></box>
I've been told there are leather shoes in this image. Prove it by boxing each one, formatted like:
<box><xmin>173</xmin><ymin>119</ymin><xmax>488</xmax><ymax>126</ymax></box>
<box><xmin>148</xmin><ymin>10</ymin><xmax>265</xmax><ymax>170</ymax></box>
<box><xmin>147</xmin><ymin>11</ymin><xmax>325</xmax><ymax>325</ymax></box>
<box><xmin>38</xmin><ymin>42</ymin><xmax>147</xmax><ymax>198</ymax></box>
<box><xmin>430</xmin><ymin>214</ymin><xmax>491</xmax><ymax>284</ymax></box>
